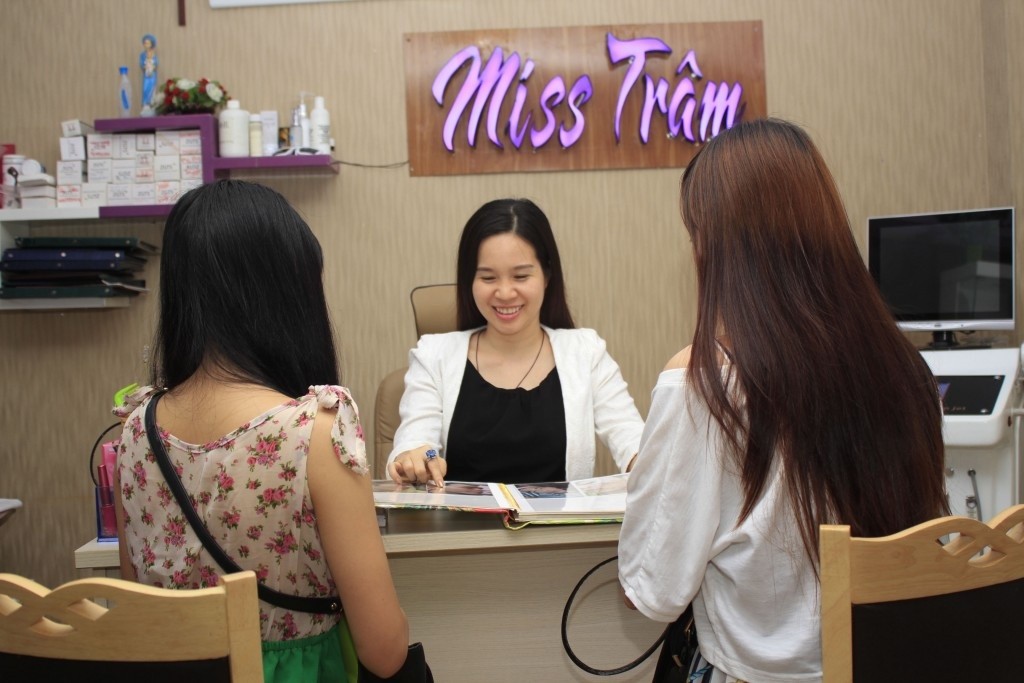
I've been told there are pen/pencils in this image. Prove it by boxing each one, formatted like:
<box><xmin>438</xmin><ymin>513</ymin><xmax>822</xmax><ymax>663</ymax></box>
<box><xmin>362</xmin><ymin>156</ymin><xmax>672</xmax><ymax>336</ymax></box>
<box><xmin>98</xmin><ymin>464</ymin><xmax>115</xmax><ymax>536</ymax></box>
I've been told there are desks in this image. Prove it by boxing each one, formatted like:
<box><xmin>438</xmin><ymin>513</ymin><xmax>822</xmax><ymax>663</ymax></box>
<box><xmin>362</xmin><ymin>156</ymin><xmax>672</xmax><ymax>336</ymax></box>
<box><xmin>72</xmin><ymin>506</ymin><xmax>669</xmax><ymax>683</ymax></box>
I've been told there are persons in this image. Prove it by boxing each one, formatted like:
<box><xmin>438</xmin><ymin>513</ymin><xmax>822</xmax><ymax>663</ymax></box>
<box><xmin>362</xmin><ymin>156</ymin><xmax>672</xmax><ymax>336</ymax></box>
<box><xmin>114</xmin><ymin>178</ymin><xmax>409</xmax><ymax>683</ymax></box>
<box><xmin>386</xmin><ymin>197</ymin><xmax>645</xmax><ymax>489</ymax></box>
<box><xmin>140</xmin><ymin>33</ymin><xmax>158</xmax><ymax>111</ymax></box>
<box><xmin>519</xmin><ymin>486</ymin><xmax>583</xmax><ymax>498</ymax></box>
<box><xmin>618</xmin><ymin>118</ymin><xmax>953</xmax><ymax>683</ymax></box>
<box><xmin>445</xmin><ymin>483</ymin><xmax>484</xmax><ymax>494</ymax></box>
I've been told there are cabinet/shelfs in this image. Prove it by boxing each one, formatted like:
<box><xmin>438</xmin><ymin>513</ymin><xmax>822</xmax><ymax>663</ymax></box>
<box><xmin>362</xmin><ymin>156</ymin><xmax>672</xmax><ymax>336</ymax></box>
<box><xmin>0</xmin><ymin>113</ymin><xmax>341</xmax><ymax>310</ymax></box>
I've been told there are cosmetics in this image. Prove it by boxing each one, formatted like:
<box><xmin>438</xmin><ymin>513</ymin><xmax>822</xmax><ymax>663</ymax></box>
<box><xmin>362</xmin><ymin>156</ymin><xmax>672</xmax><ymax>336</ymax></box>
<box><xmin>219</xmin><ymin>95</ymin><xmax>330</xmax><ymax>157</ymax></box>
<box><xmin>3</xmin><ymin>120</ymin><xmax>203</xmax><ymax>208</ymax></box>
<box><xmin>98</xmin><ymin>440</ymin><xmax>118</xmax><ymax>537</ymax></box>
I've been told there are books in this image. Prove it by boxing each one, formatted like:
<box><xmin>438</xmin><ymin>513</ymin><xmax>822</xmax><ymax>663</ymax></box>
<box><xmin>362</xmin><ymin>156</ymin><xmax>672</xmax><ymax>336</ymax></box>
<box><xmin>0</xmin><ymin>237</ymin><xmax>159</xmax><ymax>298</ymax></box>
<box><xmin>370</xmin><ymin>472</ymin><xmax>635</xmax><ymax>529</ymax></box>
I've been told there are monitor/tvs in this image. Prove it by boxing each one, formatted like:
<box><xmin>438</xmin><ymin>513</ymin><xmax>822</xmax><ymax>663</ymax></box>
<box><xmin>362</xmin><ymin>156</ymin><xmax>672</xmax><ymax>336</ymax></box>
<box><xmin>865</xmin><ymin>206</ymin><xmax>1014</xmax><ymax>347</ymax></box>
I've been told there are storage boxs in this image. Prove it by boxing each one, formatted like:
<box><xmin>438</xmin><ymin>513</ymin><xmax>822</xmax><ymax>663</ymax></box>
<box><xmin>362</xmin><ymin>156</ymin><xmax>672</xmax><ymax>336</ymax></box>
<box><xmin>18</xmin><ymin>119</ymin><xmax>204</xmax><ymax>209</ymax></box>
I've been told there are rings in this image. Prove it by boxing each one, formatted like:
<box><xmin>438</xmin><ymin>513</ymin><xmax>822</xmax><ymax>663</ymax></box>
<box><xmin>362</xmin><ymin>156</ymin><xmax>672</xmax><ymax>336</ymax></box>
<box><xmin>425</xmin><ymin>449</ymin><xmax>437</xmax><ymax>465</ymax></box>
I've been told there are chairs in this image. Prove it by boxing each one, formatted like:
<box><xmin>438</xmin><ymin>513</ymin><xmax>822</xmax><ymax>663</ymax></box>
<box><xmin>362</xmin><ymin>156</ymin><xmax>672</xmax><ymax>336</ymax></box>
<box><xmin>0</xmin><ymin>570</ymin><xmax>265</xmax><ymax>683</ymax></box>
<box><xmin>376</xmin><ymin>284</ymin><xmax>1024</xmax><ymax>683</ymax></box>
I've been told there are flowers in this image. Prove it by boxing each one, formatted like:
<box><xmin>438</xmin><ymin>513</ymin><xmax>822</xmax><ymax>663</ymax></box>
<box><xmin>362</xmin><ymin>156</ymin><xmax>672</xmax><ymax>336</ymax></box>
<box><xmin>149</xmin><ymin>76</ymin><xmax>233</xmax><ymax>116</ymax></box>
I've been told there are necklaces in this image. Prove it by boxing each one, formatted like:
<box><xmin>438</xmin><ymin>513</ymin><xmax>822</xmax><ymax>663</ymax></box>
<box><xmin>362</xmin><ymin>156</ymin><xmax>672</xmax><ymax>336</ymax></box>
<box><xmin>475</xmin><ymin>328</ymin><xmax>544</xmax><ymax>388</ymax></box>
